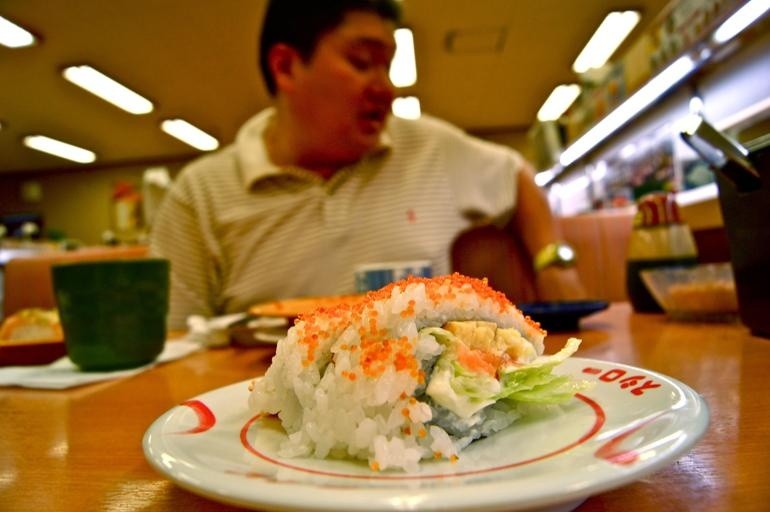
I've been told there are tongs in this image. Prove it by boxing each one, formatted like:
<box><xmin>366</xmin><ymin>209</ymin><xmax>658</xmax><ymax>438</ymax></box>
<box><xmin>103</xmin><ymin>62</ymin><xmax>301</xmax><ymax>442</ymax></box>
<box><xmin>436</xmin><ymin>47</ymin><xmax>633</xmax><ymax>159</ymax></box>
<box><xmin>676</xmin><ymin>117</ymin><xmax>762</xmax><ymax>182</ymax></box>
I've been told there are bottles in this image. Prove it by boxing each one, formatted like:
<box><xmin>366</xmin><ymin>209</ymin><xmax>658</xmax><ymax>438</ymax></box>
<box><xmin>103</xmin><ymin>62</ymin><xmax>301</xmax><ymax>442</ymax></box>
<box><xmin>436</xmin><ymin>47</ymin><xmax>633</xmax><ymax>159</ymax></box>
<box><xmin>626</xmin><ymin>191</ymin><xmax>698</xmax><ymax>314</ymax></box>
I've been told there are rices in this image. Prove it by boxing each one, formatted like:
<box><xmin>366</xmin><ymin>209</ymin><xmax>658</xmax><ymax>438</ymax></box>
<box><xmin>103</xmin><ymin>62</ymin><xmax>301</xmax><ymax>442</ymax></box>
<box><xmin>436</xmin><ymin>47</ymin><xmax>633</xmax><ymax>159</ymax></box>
<box><xmin>248</xmin><ymin>270</ymin><xmax>549</xmax><ymax>476</ymax></box>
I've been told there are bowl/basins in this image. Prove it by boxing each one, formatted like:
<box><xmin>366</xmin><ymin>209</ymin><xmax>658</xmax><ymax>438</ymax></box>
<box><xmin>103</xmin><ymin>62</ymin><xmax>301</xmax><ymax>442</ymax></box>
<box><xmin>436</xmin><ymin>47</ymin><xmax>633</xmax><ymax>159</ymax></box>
<box><xmin>641</xmin><ymin>262</ymin><xmax>737</xmax><ymax>323</ymax></box>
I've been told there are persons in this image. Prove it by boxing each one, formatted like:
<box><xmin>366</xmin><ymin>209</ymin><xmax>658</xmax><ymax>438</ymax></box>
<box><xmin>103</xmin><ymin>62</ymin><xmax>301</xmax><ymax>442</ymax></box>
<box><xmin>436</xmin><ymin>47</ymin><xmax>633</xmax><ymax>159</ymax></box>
<box><xmin>148</xmin><ymin>1</ymin><xmax>590</xmax><ymax>333</ymax></box>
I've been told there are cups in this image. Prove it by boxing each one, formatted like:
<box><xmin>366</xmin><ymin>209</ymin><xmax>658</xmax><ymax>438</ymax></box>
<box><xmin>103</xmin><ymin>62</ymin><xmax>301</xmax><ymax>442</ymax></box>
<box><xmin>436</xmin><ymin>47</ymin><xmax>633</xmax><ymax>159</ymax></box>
<box><xmin>49</xmin><ymin>256</ymin><xmax>169</xmax><ymax>372</ymax></box>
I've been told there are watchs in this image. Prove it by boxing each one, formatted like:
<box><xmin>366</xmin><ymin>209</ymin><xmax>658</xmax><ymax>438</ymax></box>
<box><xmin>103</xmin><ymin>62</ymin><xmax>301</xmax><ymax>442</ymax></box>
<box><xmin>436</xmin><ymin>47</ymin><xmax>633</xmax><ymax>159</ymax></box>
<box><xmin>533</xmin><ymin>239</ymin><xmax>577</xmax><ymax>272</ymax></box>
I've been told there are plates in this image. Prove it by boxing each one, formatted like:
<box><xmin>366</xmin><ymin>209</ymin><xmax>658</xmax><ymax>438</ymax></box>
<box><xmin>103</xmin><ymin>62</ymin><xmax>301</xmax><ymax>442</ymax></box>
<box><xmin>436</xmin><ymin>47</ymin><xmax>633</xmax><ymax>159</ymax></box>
<box><xmin>251</xmin><ymin>298</ymin><xmax>367</xmax><ymax>344</ymax></box>
<box><xmin>141</xmin><ymin>356</ymin><xmax>709</xmax><ymax>512</ymax></box>
<box><xmin>516</xmin><ymin>296</ymin><xmax>609</xmax><ymax>332</ymax></box>
